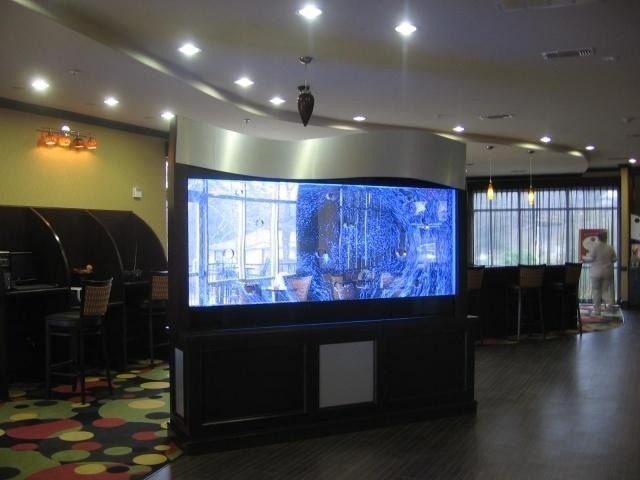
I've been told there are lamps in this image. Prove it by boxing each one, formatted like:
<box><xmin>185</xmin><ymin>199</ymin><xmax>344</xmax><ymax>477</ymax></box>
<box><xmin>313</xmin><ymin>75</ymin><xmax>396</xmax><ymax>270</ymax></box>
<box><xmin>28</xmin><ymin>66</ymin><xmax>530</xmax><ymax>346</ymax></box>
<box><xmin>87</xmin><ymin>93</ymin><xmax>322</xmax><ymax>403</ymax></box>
<box><xmin>38</xmin><ymin>127</ymin><xmax>98</xmax><ymax>150</ymax></box>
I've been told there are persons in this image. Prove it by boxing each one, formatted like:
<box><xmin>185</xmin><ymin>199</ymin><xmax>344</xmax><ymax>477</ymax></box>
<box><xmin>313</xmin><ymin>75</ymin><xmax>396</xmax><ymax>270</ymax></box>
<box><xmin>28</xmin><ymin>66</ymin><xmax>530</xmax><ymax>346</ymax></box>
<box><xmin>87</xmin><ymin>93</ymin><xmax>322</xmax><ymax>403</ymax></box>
<box><xmin>581</xmin><ymin>230</ymin><xmax>619</xmax><ymax>316</ymax></box>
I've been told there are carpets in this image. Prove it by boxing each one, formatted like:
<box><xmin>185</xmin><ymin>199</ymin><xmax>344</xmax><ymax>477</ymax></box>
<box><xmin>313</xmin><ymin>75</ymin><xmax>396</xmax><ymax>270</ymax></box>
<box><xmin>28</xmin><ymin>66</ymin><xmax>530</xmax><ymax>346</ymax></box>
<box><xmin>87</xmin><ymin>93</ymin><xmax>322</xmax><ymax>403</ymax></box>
<box><xmin>0</xmin><ymin>356</ymin><xmax>188</xmax><ymax>479</ymax></box>
<box><xmin>474</xmin><ymin>302</ymin><xmax>625</xmax><ymax>344</ymax></box>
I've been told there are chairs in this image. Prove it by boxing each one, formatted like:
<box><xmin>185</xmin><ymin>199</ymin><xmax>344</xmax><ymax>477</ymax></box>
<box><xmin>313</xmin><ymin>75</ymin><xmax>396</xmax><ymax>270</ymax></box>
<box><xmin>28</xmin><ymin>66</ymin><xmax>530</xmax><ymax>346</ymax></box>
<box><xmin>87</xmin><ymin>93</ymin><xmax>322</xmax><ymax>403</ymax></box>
<box><xmin>43</xmin><ymin>270</ymin><xmax>169</xmax><ymax>405</ymax></box>
<box><xmin>467</xmin><ymin>262</ymin><xmax>583</xmax><ymax>343</ymax></box>
